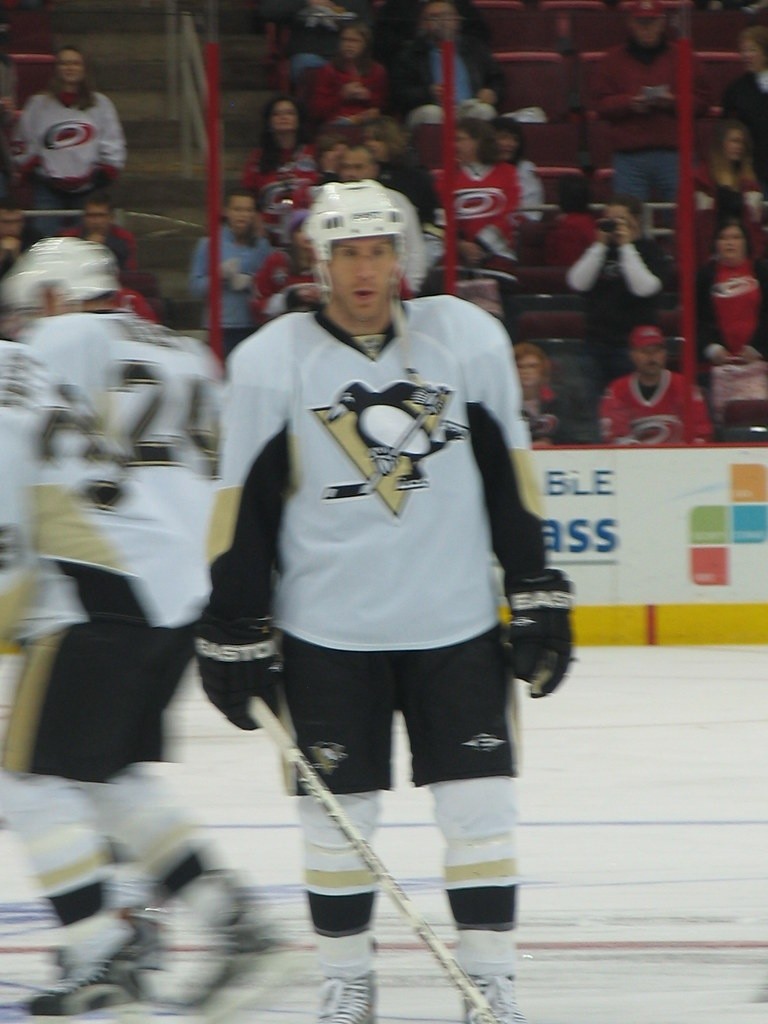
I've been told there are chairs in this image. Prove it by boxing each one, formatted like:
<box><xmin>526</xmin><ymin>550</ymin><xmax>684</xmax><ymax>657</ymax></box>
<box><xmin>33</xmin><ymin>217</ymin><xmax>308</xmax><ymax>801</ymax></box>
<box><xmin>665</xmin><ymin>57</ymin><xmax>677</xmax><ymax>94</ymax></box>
<box><xmin>469</xmin><ymin>0</ymin><xmax>744</xmax><ymax>359</ymax></box>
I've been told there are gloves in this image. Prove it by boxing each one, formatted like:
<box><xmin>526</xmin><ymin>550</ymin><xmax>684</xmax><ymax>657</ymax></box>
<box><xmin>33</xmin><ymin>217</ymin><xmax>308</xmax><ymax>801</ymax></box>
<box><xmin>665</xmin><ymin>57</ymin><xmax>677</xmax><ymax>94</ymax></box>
<box><xmin>192</xmin><ymin>609</ymin><xmax>278</xmax><ymax>731</ymax></box>
<box><xmin>503</xmin><ymin>570</ymin><xmax>573</xmax><ymax>699</ymax></box>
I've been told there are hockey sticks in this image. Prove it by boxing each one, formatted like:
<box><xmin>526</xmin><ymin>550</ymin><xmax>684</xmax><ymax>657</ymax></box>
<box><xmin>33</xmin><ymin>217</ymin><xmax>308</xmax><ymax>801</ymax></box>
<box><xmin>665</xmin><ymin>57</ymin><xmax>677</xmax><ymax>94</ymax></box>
<box><xmin>247</xmin><ymin>696</ymin><xmax>521</xmax><ymax>1024</ymax></box>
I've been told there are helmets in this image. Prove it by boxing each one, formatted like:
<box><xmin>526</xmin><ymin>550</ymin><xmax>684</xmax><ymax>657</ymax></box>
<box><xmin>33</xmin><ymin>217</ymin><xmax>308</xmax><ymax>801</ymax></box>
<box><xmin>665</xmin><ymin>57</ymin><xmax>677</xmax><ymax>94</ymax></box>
<box><xmin>409</xmin><ymin>105</ymin><xmax>444</xmax><ymax>127</ymax></box>
<box><xmin>2</xmin><ymin>239</ymin><xmax>122</xmax><ymax>310</ymax></box>
<box><xmin>299</xmin><ymin>179</ymin><xmax>407</xmax><ymax>248</ymax></box>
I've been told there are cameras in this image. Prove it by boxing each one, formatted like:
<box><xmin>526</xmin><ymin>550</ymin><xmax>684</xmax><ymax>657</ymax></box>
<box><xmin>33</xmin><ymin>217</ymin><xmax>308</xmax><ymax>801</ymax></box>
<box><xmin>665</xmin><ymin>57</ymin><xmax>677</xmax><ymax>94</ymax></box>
<box><xmin>598</xmin><ymin>220</ymin><xmax>617</xmax><ymax>233</ymax></box>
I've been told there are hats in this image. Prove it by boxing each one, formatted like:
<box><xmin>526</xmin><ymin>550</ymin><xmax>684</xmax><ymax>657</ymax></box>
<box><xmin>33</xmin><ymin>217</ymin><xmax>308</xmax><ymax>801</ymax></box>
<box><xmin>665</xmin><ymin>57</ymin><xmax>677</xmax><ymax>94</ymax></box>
<box><xmin>466</xmin><ymin>103</ymin><xmax>497</xmax><ymax>122</ymax></box>
<box><xmin>632</xmin><ymin>0</ymin><xmax>665</xmax><ymax>19</ymax></box>
<box><xmin>631</xmin><ymin>326</ymin><xmax>665</xmax><ymax>349</ymax></box>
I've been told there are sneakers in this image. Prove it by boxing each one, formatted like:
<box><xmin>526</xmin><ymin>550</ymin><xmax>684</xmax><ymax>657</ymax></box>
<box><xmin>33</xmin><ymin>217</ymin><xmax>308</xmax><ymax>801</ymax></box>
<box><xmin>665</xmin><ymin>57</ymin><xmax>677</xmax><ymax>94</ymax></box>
<box><xmin>464</xmin><ymin>974</ymin><xmax>528</xmax><ymax>1024</ymax></box>
<box><xmin>314</xmin><ymin>971</ymin><xmax>375</xmax><ymax>1024</ymax></box>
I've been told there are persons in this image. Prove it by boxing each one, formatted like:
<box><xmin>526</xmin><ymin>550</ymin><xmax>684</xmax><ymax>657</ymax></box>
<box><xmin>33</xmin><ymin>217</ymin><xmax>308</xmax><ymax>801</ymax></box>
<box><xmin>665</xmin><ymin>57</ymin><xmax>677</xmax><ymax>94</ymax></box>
<box><xmin>0</xmin><ymin>0</ymin><xmax>768</xmax><ymax>1024</ymax></box>
<box><xmin>195</xmin><ymin>177</ymin><xmax>575</xmax><ymax>1024</ymax></box>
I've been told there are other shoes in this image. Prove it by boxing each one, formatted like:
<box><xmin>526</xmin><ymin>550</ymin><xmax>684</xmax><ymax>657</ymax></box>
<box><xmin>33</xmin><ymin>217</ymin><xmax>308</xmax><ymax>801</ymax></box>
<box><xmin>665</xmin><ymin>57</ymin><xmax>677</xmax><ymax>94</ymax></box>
<box><xmin>28</xmin><ymin>920</ymin><xmax>151</xmax><ymax>1023</ymax></box>
<box><xmin>168</xmin><ymin>909</ymin><xmax>274</xmax><ymax>1024</ymax></box>
